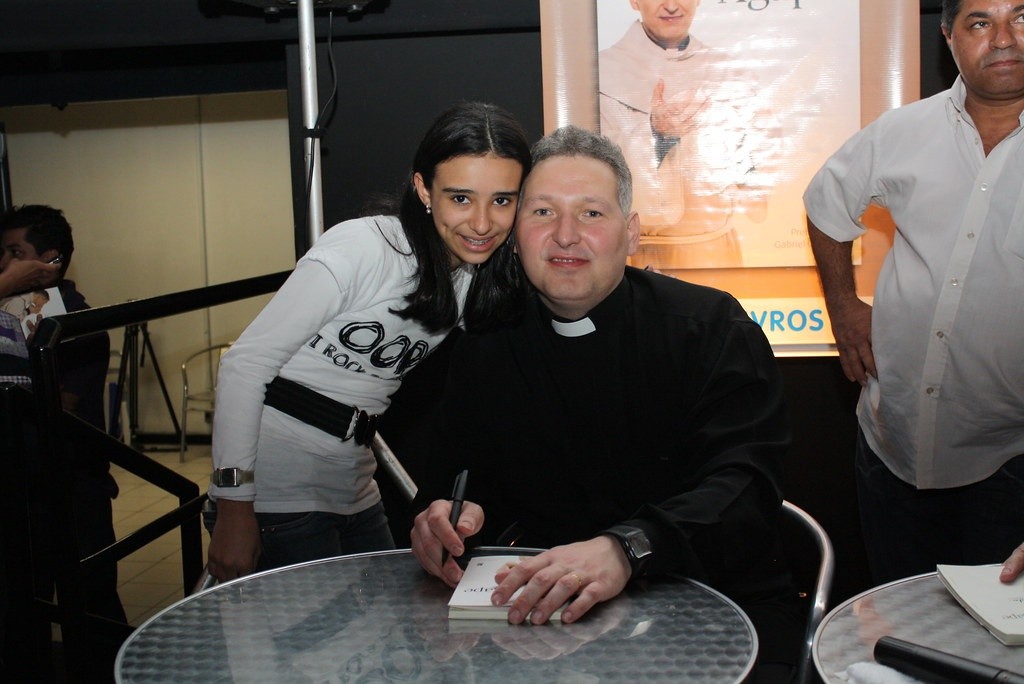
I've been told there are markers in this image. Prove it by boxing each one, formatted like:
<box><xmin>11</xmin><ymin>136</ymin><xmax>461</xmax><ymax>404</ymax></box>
<box><xmin>873</xmin><ymin>634</ymin><xmax>1024</xmax><ymax>684</ymax></box>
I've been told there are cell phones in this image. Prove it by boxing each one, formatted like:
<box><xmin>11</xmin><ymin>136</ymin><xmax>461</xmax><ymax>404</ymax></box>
<box><xmin>48</xmin><ymin>254</ymin><xmax>64</xmax><ymax>266</ymax></box>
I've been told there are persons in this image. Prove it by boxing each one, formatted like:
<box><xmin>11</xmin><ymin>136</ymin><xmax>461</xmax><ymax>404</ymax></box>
<box><xmin>802</xmin><ymin>0</ymin><xmax>1024</xmax><ymax>581</ymax></box>
<box><xmin>0</xmin><ymin>205</ymin><xmax>128</xmax><ymax>684</ymax></box>
<box><xmin>599</xmin><ymin>0</ymin><xmax>744</xmax><ymax>267</ymax></box>
<box><xmin>26</xmin><ymin>291</ymin><xmax>49</xmax><ymax>316</ymax></box>
<box><xmin>202</xmin><ymin>101</ymin><xmax>532</xmax><ymax>584</ymax></box>
<box><xmin>375</xmin><ymin>126</ymin><xmax>792</xmax><ymax>626</ymax></box>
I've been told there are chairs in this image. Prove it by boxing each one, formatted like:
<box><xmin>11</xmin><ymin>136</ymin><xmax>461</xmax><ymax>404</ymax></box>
<box><xmin>781</xmin><ymin>499</ymin><xmax>837</xmax><ymax>684</ymax></box>
<box><xmin>180</xmin><ymin>342</ymin><xmax>233</xmax><ymax>461</ymax></box>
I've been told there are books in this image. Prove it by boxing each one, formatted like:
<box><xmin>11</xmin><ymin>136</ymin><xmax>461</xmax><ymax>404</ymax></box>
<box><xmin>936</xmin><ymin>564</ymin><xmax>1024</xmax><ymax>645</ymax></box>
<box><xmin>447</xmin><ymin>556</ymin><xmax>569</xmax><ymax>620</ymax></box>
<box><xmin>21</xmin><ymin>287</ymin><xmax>74</xmax><ymax>343</ymax></box>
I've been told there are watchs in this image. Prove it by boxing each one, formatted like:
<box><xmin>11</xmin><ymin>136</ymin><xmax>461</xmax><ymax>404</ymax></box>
<box><xmin>213</xmin><ymin>468</ymin><xmax>254</xmax><ymax>487</ymax></box>
<box><xmin>603</xmin><ymin>525</ymin><xmax>654</xmax><ymax>571</ymax></box>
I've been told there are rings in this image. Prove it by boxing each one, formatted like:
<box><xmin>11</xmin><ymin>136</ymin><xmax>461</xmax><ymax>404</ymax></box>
<box><xmin>569</xmin><ymin>573</ymin><xmax>582</xmax><ymax>585</ymax></box>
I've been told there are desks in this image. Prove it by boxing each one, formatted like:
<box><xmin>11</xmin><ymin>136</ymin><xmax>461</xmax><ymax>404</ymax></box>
<box><xmin>115</xmin><ymin>545</ymin><xmax>759</xmax><ymax>684</ymax></box>
<box><xmin>812</xmin><ymin>563</ymin><xmax>1024</xmax><ymax>684</ymax></box>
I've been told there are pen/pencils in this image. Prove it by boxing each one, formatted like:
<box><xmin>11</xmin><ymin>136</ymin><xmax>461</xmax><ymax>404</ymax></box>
<box><xmin>440</xmin><ymin>469</ymin><xmax>469</xmax><ymax>568</ymax></box>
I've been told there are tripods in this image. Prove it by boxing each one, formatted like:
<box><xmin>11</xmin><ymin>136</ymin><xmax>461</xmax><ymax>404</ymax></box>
<box><xmin>110</xmin><ymin>321</ymin><xmax>188</xmax><ymax>455</ymax></box>
